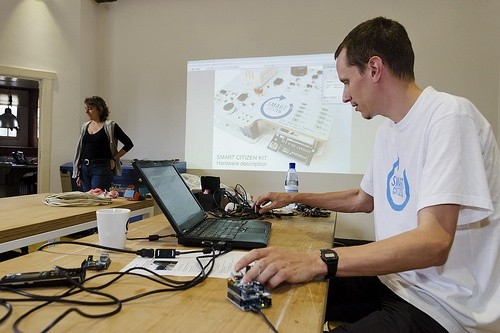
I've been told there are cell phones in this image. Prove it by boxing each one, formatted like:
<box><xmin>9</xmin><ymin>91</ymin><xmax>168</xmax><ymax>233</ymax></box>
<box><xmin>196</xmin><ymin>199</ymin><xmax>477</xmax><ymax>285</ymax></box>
<box><xmin>0</xmin><ymin>268</ymin><xmax>87</xmax><ymax>291</ymax></box>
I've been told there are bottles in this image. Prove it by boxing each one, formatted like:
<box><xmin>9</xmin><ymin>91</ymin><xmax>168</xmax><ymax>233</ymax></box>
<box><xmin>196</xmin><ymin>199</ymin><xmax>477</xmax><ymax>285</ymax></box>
<box><xmin>284</xmin><ymin>162</ymin><xmax>299</xmax><ymax>209</ymax></box>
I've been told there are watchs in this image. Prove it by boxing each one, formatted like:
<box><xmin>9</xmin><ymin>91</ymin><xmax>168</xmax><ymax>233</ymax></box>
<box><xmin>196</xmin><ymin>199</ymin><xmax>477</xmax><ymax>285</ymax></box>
<box><xmin>320</xmin><ymin>248</ymin><xmax>339</xmax><ymax>278</ymax></box>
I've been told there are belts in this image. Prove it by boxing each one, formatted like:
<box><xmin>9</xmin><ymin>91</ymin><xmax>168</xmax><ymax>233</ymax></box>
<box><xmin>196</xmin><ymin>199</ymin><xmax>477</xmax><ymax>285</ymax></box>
<box><xmin>80</xmin><ymin>158</ymin><xmax>110</xmax><ymax>166</ymax></box>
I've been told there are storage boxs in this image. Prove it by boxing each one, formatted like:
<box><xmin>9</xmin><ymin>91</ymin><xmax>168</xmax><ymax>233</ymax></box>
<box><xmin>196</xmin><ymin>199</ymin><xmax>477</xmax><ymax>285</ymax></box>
<box><xmin>193</xmin><ymin>176</ymin><xmax>229</xmax><ymax>211</ymax></box>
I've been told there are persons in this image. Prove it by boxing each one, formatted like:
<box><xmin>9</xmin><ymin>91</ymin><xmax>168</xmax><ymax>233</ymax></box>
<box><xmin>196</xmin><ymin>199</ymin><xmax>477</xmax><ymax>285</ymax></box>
<box><xmin>234</xmin><ymin>16</ymin><xmax>500</xmax><ymax>333</ymax></box>
<box><xmin>72</xmin><ymin>96</ymin><xmax>133</xmax><ymax>192</ymax></box>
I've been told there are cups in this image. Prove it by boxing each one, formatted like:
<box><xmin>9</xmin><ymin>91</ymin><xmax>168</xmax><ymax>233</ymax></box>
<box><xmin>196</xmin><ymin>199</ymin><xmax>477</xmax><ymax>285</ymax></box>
<box><xmin>96</xmin><ymin>208</ymin><xmax>131</xmax><ymax>252</ymax></box>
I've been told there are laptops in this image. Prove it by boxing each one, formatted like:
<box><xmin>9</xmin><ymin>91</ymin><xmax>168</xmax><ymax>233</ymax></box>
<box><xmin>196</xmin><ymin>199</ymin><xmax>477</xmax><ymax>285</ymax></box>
<box><xmin>132</xmin><ymin>160</ymin><xmax>271</xmax><ymax>249</ymax></box>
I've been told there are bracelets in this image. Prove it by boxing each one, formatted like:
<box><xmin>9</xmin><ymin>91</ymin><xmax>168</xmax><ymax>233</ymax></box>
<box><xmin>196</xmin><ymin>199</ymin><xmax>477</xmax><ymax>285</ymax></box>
<box><xmin>77</xmin><ymin>176</ymin><xmax>79</xmax><ymax>177</ymax></box>
<box><xmin>112</xmin><ymin>158</ymin><xmax>116</xmax><ymax>161</ymax></box>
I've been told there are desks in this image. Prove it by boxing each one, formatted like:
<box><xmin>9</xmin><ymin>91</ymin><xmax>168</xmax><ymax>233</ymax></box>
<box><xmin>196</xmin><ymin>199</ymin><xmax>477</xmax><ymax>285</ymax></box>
<box><xmin>0</xmin><ymin>211</ymin><xmax>337</xmax><ymax>332</ymax></box>
<box><xmin>0</xmin><ymin>162</ymin><xmax>39</xmax><ymax>197</ymax></box>
<box><xmin>0</xmin><ymin>193</ymin><xmax>154</xmax><ymax>254</ymax></box>
<box><xmin>59</xmin><ymin>159</ymin><xmax>188</xmax><ymax>194</ymax></box>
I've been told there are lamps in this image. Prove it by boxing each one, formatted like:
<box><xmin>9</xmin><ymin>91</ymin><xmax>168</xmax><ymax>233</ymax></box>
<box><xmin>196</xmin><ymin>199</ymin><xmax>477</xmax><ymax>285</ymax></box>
<box><xmin>0</xmin><ymin>86</ymin><xmax>18</xmax><ymax>129</ymax></box>
